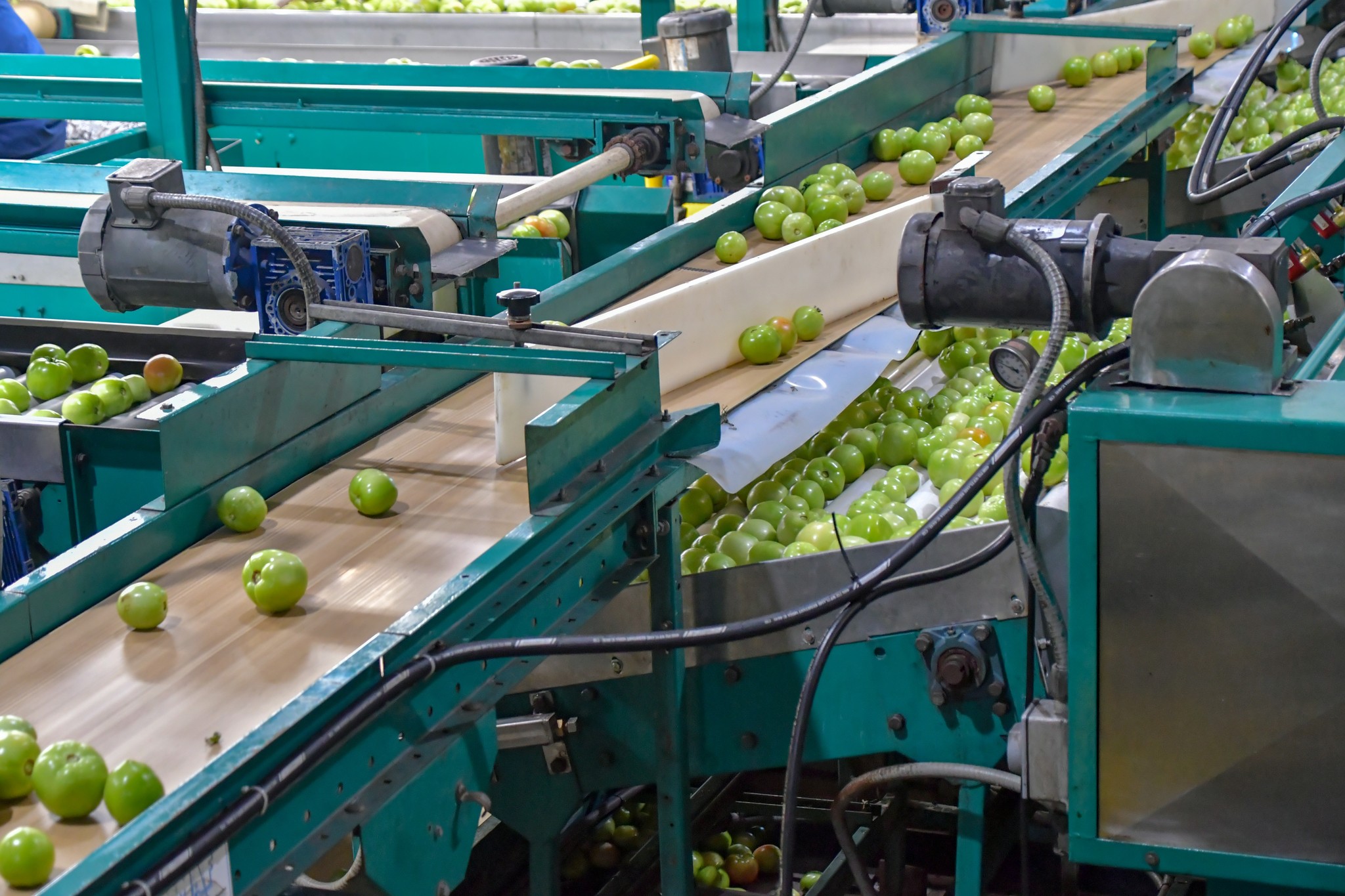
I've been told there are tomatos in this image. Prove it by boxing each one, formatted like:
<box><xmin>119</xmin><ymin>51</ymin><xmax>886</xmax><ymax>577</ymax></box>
<box><xmin>117</xmin><ymin>580</ymin><xmax>168</xmax><ymax>630</ymax></box>
<box><xmin>242</xmin><ymin>549</ymin><xmax>308</xmax><ymax>611</ymax></box>
<box><xmin>111</xmin><ymin>0</ymin><xmax>1345</xmax><ymax>268</ymax></box>
<box><xmin>217</xmin><ymin>486</ymin><xmax>268</xmax><ymax>532</ymax></box>
<box><xmin>74</xmin><ymin>44</ymin><xmax>101</xmax><ymax>56</ymax></box>
<box><xmin>349</xmin><ymin>468</ymin><xmax>398</xmax><ymax>515</ymax></box>
<box><xmin>0</xmin><ymin>714</ymin><xmax>165</xmax><ymax>888</ymax></box>
<box><xmin>632</xmin><ymin>303</ymin><xmax>1291</xmax><ymax>587</ymax></box>
<box><xmin>0</xmin><ymin>342</ymin><xmax>183</xmax><ymax>424</ymax></box>
<box><xmin>562</xmin><ymin>796</ymin><xmax>824</xmax><ymax>896</ymax></box>
